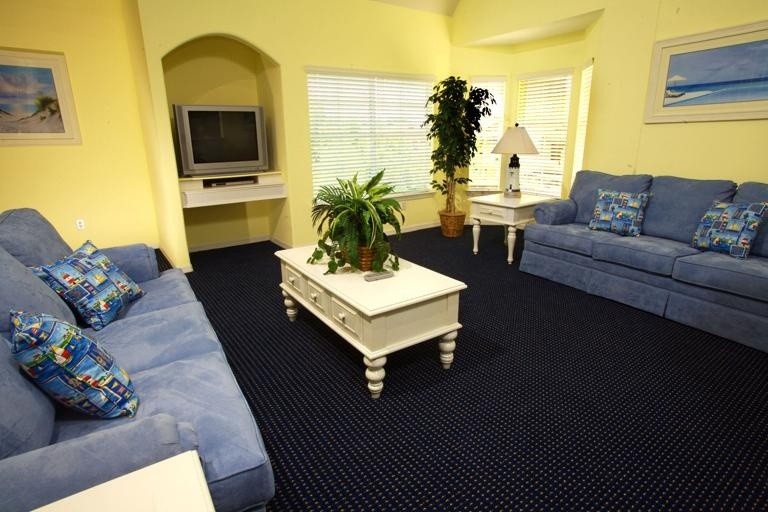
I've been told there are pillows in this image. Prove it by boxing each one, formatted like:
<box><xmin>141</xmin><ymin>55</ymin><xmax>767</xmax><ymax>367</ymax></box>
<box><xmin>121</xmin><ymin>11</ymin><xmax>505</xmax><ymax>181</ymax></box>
<box><xmin>588</xmin><ymin>187</ymin><xmax>655</xmax><ymax>239</ymax></box>
<box><xmin>27</xmin><ymin>239</ymin><xmax>148</xmax><ymax>331</ymax></box>
<box><xmin>7</xmin><ymin>307</ymin><xmax>146</xmax><ymax>420</ymax></box>
<box><xmin>688</xmin><ymin>199</ymin><xmax>768</xmax><ymax>260</ymax></box>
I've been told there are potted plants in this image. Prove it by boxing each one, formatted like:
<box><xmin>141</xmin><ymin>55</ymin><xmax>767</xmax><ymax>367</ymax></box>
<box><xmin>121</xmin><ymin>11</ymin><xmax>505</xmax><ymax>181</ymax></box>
<box><xmin>306</xmin><ymin>167</ymin><xmax>406</xmax><ymax>275</ymax></box>
<box><xmin>420</xmin><ymin>76</ymin><xmax>498</xmax><ymax>238</ymax></box>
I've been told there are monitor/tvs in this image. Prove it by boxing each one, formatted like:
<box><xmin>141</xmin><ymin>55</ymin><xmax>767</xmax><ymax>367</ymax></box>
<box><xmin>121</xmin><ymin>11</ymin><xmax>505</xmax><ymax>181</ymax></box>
<box><xmin>172</xmin><ymin>104</ymin><xmax>269</xmax><ymax>176</ymax></box>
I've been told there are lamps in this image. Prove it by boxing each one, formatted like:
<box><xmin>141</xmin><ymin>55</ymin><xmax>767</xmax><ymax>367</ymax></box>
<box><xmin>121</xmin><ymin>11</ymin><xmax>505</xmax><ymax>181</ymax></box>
<box><xmin>490</xmin><ymin>122</ymin><xmax>539</xmax><ymax>197</ymax></box>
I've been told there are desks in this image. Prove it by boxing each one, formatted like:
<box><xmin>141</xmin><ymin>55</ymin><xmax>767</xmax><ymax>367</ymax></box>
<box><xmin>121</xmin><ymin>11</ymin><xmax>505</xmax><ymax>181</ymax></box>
<box><xmin>274</xmin><ymin>242</ymin><xmax>468</xmax><ymax>398</ymax></box>
<box><xmin>467</xmin><ymin>192</ymin><xmax>554</xmax><ymax>265</ymax></box>
<box><xmin>31</xmin><ymin>449</ymin><xmax>216</xmax><ymax>512</ymax></box>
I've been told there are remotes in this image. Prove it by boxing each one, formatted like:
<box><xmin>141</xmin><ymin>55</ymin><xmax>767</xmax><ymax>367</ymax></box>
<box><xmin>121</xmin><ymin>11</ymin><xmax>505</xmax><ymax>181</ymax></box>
<box><xmin>364</xmin><ymin>271</ymin><xmax>394</xmax><ymax>281</ymax></box>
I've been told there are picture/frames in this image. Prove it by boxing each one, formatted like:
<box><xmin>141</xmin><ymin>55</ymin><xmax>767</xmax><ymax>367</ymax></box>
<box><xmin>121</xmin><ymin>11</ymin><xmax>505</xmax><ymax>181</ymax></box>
<box><xmin>643</xmin><ymin>20</ymin><xmax>768</xmax><ymax>125</ymax></box>
<box><xmin>0</xmin><ymin>48</ymin><xmax>83</xmax><ymax>145</ymax></box>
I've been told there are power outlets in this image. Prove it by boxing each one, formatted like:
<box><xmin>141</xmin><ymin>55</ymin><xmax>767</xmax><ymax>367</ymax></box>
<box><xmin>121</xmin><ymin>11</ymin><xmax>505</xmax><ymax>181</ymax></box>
<box><xmin>76</xmin><ymin>218</ymin><xmax>84</xmax><ymax>229</ymax></box>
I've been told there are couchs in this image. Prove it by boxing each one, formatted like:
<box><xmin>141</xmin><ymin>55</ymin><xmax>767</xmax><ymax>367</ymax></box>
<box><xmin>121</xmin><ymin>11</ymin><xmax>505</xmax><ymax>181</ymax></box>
<box><xmin>0</xmin><ymin>208</ymin><xmax>276</xmax><ymax>512</ymax></box>
<box><xmin>518</xmin><ymin>170</ymin><xmax>768</xmax><ymax>354</ymax></box>
